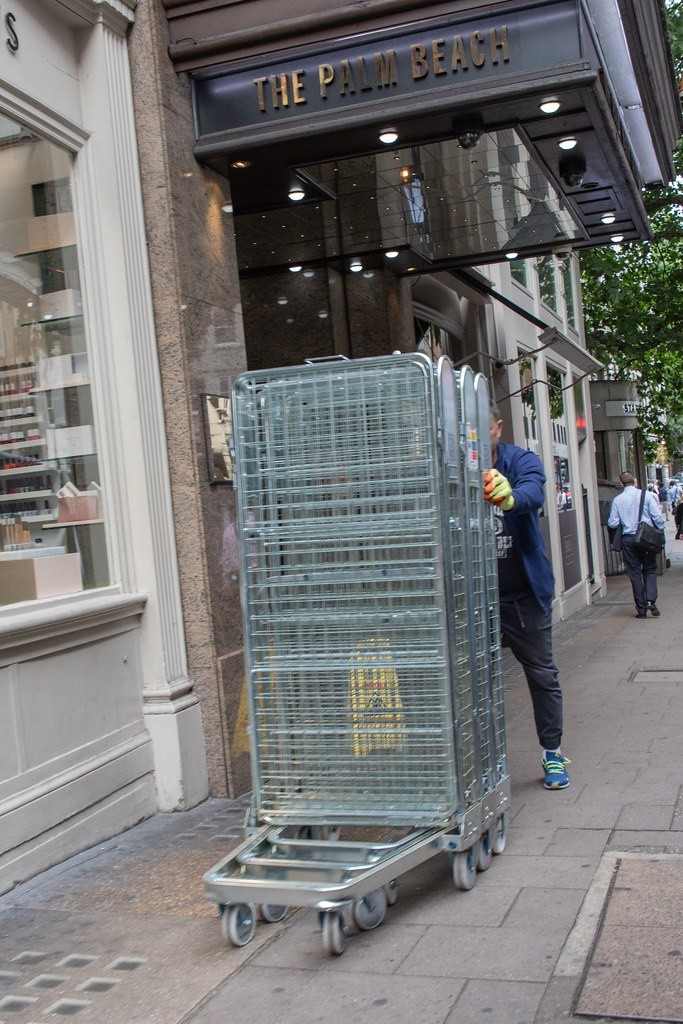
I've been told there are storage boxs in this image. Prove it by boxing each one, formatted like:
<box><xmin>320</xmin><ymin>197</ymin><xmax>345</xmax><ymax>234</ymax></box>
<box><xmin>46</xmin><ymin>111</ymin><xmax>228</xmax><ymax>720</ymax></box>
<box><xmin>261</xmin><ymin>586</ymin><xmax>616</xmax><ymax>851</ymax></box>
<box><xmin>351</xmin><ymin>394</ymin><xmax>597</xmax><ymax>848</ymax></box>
<box><xmin>38</xmin><ymin>289</ymin><xmax>84</xmax><ymax>320</ymax></box>
<box><xmin>0</xmin><ymin>552</ymin><xmax>84</xmax><ymax>606</ymax></box>
<box><xmin>27</xmin><ymin>212</ymin><xmax>75</xmax><ymax>251</ymax></box>
<box><xmin>47</xmin><ymin>425</ymin><xmax>97</xmax><ymax>458</ymax></box>
<box><xmin>39</xmin><ymin>352</ymin><xmax>90</xmax><ymax>387</ymax></box>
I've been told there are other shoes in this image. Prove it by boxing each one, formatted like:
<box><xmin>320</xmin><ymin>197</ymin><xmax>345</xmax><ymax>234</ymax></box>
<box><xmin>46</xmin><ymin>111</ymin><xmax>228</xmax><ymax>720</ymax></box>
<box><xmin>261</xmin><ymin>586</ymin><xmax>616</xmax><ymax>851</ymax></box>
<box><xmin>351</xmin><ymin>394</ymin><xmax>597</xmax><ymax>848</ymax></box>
<box><xmin>645</xmin><ymin>601</ymin><xmax>660</xmax><ymax>617</ymax></box>
<box><xmin>634</xmin><ymin>614</ymin><xmax>645</xmax><ymax>619</ymax></box>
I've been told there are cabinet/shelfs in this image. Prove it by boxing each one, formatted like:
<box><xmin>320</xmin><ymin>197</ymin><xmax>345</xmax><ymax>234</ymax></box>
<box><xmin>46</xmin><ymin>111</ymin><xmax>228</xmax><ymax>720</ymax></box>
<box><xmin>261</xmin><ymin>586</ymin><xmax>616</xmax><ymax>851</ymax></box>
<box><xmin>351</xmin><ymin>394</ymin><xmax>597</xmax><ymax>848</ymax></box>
<box><xmin>13</xmin><ymin>240</ymin><xmax>109</xmax><ymax>531</ymax></box>
<box><xmin>0</xmin><ymin>366</ymin><xmax>68</xmax><ymax>558</ymax></box>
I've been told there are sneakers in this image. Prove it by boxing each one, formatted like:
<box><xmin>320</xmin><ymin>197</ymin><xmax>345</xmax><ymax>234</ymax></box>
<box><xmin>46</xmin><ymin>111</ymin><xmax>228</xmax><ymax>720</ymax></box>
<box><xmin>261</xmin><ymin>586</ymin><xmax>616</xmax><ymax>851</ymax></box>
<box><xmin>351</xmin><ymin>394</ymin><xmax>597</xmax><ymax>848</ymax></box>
<box><xmin>540</xmin><ymin>751</ymin><xmax>571</xmax><ymax>789</ymax></box>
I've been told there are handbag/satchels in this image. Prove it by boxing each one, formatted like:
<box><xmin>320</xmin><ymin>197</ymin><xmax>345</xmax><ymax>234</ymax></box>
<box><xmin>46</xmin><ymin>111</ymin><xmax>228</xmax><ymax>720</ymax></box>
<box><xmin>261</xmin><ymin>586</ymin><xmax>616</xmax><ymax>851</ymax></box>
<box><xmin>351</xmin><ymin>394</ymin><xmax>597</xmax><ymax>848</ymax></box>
<box><xmin>634</xmin><ymin>520</ymin><xmax>663</xmax><ymax>555</ymax></box>
<box><xmin>671</xmin><ymin>507</ymin><xmax>676</xmax><ymax>515</ymax></box>
<box><xmin>658</xmin><ymin>493</ymin><xmax>667</xmax><ymax>502</ymax></box>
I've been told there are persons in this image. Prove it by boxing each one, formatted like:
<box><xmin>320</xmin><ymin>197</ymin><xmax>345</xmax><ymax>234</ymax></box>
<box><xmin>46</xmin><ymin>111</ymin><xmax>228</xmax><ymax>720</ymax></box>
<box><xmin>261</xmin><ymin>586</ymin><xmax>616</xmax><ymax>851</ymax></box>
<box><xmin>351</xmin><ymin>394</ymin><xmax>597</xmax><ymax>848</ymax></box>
<box><xmin>480</xmin><ymin>401</ymin><xmax>571</xmax><ymax>791</ymax></box>
<box><xmin>607</xmin><ymin>471</ymin><xmax>667</xmax><ymax>620</ymax></box>
<box><xmin>647</xmin><ymin>478</ymin><xmax>683</xmax><ymax>540</ymax></box>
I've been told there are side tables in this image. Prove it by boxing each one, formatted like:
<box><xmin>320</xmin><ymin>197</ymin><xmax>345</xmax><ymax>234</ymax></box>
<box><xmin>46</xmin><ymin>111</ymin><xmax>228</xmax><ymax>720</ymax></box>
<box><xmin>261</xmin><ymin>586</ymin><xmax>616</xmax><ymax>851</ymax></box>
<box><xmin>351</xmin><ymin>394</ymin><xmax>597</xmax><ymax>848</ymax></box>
<box><xmin>58</xmin><ymin>495</ymin><xmax>96</xmax><ymax>523</ymax></box>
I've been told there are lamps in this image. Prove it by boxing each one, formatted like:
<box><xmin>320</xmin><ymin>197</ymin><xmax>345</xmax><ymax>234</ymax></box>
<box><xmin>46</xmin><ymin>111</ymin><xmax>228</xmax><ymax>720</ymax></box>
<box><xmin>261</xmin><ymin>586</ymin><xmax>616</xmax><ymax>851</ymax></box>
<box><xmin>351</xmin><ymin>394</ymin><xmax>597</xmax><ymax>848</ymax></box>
<box><xmin>380</xmin><ymin>129</ymin><xmax>398</xmax><ymax>144</ymax></box>
<box><xmin>600</xmin><ymin>214</ymin><xmax>616</xmax><ymax>224</ymax></box>
<box><xmin>504</xmin><ymin>251</ymin><xmax>520</xmax><ymax>261</ymax></box>
<box><xmin>351</xmin><ymin>261</ymin><xmax>363</xmax><ymax>274</ymax></box>
<box><xmin>289</xmin><ymin>264</ymin><xmax>301</xmax><ymax>272</ymax></box>
<box><xmin>385</xmin><ymin>251</ymin><xmax>401</xmax><ymax>258</ymax></box>
<box><xmin>610</xmin><ymin>233</ymin><xmax>624</xmax><ymax>243</ymax></box>
<box><xmin>289</xmin><ymin>187</ymin><xmax>305</xmax><ymax>201</ymax></box>
<box><xmin>557</xmin><ymin>136</ymin><xmax>577</xmax><ymax>149</ymax></box>
<box><xmin>539</xmin><ymin>97</ymin><xmax>562</xmax><ymax>113</ymax></box>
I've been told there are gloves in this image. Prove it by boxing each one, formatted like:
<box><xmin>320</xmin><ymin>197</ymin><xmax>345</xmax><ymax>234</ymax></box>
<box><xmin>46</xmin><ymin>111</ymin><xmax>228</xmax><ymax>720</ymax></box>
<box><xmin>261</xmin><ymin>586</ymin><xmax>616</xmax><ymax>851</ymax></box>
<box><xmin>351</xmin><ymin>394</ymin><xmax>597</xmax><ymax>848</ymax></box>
<box><xmin>482</xmin><ymin>469</ymin><xmax>514</xmax><ymax>511</ymax></box>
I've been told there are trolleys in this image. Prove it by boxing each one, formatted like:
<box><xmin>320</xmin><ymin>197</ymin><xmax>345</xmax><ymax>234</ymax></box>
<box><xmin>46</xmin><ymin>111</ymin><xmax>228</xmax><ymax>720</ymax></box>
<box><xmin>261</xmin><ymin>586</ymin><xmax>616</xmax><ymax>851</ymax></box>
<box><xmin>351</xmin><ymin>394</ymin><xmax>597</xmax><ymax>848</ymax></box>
<box><xmin>203</xmin><ymin>350</ymin><xmax>515</xmax><ymax>954</ymax></box>
